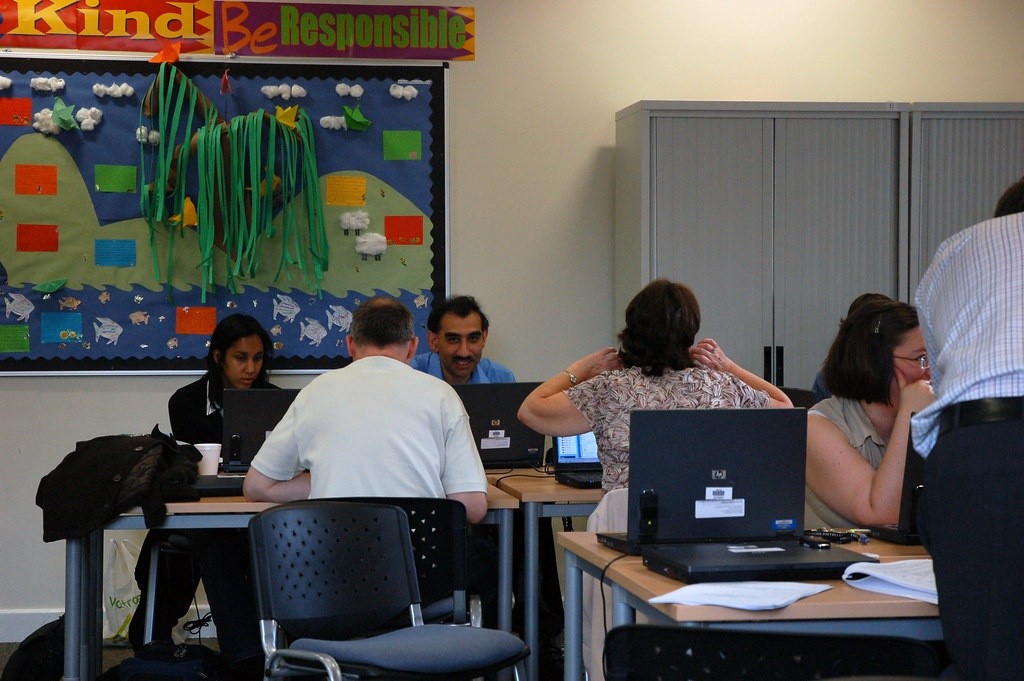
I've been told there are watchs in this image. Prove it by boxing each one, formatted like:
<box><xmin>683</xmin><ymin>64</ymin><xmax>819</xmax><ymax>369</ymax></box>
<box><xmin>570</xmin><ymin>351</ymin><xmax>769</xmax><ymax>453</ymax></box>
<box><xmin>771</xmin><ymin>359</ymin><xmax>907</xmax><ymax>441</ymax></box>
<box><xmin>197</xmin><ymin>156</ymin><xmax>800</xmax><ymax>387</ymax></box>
<box><xmin>564</xmin><ymin>368</ymin><xmax>577</xmax><ymax>384</ymax></box>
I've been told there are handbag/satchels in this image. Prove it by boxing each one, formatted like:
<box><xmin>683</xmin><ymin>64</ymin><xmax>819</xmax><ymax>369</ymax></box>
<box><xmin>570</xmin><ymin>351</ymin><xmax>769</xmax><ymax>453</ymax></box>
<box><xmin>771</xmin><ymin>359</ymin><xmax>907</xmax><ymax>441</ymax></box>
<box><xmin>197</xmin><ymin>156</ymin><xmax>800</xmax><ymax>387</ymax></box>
<box><xmin>104</xmin><ymin>539</ymin><xmax>197</xmax><ymax>646</ymax></box>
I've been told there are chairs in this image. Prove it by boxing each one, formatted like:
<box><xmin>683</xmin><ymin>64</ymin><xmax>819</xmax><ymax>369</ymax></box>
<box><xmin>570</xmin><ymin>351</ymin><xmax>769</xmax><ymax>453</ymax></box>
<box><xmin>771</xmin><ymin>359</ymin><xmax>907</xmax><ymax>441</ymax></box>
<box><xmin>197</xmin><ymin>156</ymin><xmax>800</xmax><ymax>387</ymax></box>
<box><xmin>603</xmin><ymin>624</ymin><xmax>936</xmax><ymax>681</ymax></box>
<box><xmin>248</xmin><ymin>495</ymin><xmax>531</xmax><ymax>681</ymax></box>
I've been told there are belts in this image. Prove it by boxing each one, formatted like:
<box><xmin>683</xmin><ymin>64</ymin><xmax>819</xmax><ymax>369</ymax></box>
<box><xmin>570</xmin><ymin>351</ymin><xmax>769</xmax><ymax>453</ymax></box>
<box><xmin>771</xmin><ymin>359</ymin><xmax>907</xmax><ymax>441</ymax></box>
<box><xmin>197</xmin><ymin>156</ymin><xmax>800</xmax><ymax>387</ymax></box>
<box><xmin>936</xmin><ymin>397</ymin><xmax>1024</xmax><ymax>438</ymax></box>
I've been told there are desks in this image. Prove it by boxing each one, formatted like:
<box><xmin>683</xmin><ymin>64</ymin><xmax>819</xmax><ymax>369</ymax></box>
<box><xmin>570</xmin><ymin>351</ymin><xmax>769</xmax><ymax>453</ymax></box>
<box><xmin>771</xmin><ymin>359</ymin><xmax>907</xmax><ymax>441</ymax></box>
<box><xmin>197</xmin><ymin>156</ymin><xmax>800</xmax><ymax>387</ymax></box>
<box><xmin>62</xmin><ymin>479</ymin><xmax>521</xmax><ymax>681</ymax></box>
<box><xmin>555</xmin><ymin>528</ymin><xmax>939</xmax><ymax>681</ymax></box>
<box><xmin>493</xmin><ymin>472</ymin><xmax>603</xmax><ymax>681</ymax></box>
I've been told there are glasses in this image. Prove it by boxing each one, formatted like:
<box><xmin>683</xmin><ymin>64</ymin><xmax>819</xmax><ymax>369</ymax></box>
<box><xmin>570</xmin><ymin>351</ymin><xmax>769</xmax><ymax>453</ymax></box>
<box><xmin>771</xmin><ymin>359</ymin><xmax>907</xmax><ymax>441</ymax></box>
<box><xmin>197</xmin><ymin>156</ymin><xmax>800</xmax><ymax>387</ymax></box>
<box><xmin>893</xmin><ymin>355</ymin><xmax>930</xmax><ymax>370</ymax></box>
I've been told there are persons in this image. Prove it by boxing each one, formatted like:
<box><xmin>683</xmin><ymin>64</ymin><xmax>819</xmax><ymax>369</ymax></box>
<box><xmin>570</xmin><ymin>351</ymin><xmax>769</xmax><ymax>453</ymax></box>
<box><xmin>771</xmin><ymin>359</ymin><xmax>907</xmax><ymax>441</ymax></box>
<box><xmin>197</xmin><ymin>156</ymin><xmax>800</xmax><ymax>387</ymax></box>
<box><xmin>243</xmin><ymin>295</ymin><xmax>488</xmax><ymax>525</ymax></box>
<box><xmin>911</xmin><ymin>179</ymin><xmax>1024</xmax><ymax>681</ymax></box>
<box><xmin>805</xmin><ymin>293</ymin><xmax>934</xmax><ymax>530</ymax></box>
<box><xmin>409</xmin><ymin>296</ymin><xmax>515</xmax><ymax>384</ymax></box>
<box><xmin>128</xmin><ymin>312</ymin><xmax>283</xmax><ymax>681</ymax></box>
<box><xmin>517</xmin><ymin>279</ymin><xmax>795</xmax><ymax>503</ymax></box>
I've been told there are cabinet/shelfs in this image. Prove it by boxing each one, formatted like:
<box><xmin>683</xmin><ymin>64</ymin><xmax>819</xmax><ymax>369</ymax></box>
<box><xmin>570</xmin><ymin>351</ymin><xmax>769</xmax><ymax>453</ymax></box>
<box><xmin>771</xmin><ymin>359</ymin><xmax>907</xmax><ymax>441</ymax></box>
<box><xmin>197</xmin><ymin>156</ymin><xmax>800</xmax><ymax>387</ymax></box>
<box><xmin>615</xmin><ymin>98</ymin><xmax>1024</xmax><ymax>392</ymax></box>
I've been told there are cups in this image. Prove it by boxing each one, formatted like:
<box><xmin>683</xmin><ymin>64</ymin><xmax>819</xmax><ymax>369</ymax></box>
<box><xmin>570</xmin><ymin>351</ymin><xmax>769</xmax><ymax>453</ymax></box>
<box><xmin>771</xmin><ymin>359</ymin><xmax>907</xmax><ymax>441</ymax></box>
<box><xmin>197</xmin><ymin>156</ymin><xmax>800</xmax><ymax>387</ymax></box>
<box><xmin>194</xmin><ymin>444</ymin><xmax>222</xmax><ymax>476</ymax></box>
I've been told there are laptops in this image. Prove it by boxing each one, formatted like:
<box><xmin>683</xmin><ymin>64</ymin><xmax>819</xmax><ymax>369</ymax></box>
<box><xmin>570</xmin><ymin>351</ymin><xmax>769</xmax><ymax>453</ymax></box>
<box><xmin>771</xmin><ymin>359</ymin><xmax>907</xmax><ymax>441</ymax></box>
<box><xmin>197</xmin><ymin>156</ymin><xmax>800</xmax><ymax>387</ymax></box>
<box><xmin>191</xmin><ymin>475</ymin><xmax>247</xmax><ymax>497</ymax></box>
<box><xmin>594</xmin><ymin>407</ymin><xmax>809</xmax><ymax>555</ymax></box>
<box><xmin>643</xmin><ymin>541</ymin><xmax>878</xmax><ymax>584</ymax></box>
<box><xmin>217</xmin><ymin>388</ymin><xmax>303</xmax><ymax>473</ymax></box>
<box><xmin>450</xmin><ymin>381</ymin><xmax>544</xmax><ymax>469</ymax></box>
<box><xmin>857</xmin><ymin>412</ymin><xmax>926</xmax><ymax>545</ymax></box>
<box><xmin>552</xmin><ymin>430</ymin><xmax>603</xmax><ymax>489</ymax></box>
